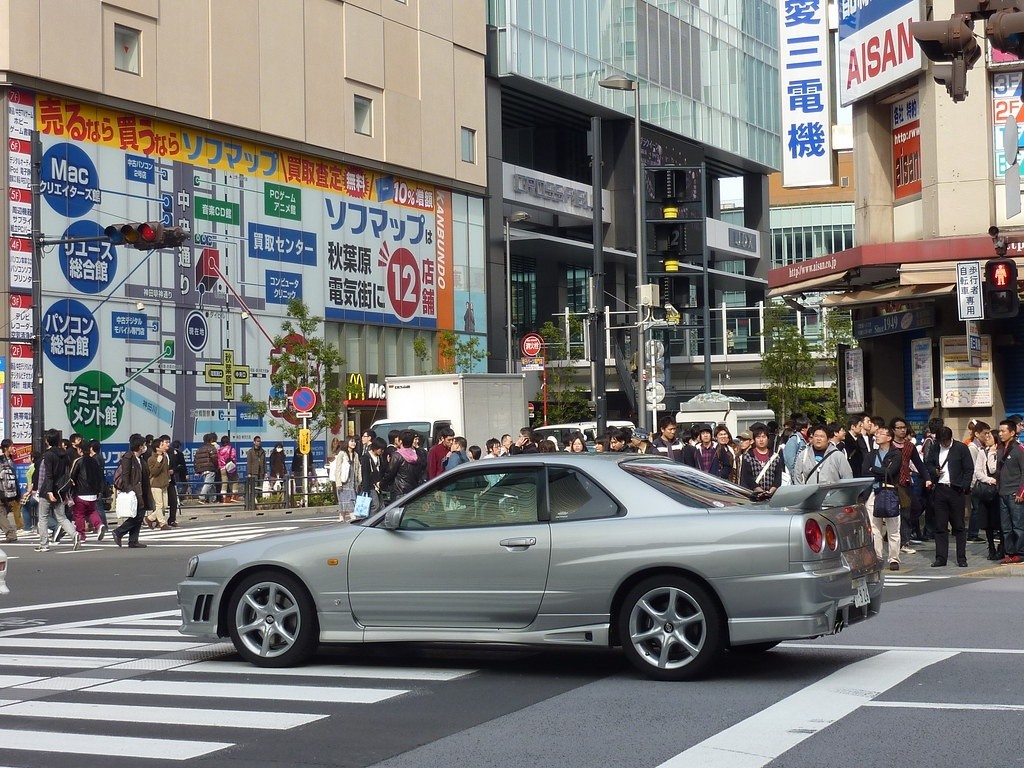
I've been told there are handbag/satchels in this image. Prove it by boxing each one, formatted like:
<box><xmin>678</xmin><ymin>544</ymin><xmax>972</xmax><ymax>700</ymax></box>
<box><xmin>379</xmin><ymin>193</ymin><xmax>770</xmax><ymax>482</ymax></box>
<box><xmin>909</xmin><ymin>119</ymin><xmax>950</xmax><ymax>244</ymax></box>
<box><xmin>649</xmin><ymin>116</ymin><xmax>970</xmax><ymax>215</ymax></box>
<box><xmin>329</xmin><ymin>452</ymin><xmax>351</xmax><ymax>483</ymax></box>
<box><xmin>116</xmin><ymin>490</ymin><xmax>137</xmax><ymax>519</ymax></box>
<box><xmin>21</xmin><ymin>493</ymin><xmax>32</xmax><ymax>505</ymax></box>
<box><xmin>972</xmin><ymin>480</ymin><xmax>995</xmax><ymax>504</ymax></box>
<box><xmin>225</xmin><ymin>458</ymin><xmax>236</xmax><ymax>474</ymax></box>
<box><xmin>263</xmin><ymin>478</ymin><xmax>272</xmax><ymax>498</ymax></box>
<box><xmin>272</xmin><ymin>476</ymin><xmax>281</xmax><ymax>490</ymax></box>
<box><xmin>924</xmin><ymin>491</ymin><xmax>935</xmax><ymax>516</ymax></box>
<box><xmin>873</xmin><ymin>468</ymin><xmax>899</xmax><ymax>517</ymax></box>
<box><xmin>113</xmin><ymin>465</ymin><xmax>124</xmax><ymax>489</ymax></box>
<box><xmin>354</xmin><ymin>492</ymin><xmax>372</xmax><ymax>518</ymax></box>
<box><xmin>781</xmin><ymin>471</ymin><xmax>794</xmax><ymax>486</ymax></box>
<box><xmin>53</xmin><ymin>475</ymin><xmax>75</xmax><ymax>503</ymax></box>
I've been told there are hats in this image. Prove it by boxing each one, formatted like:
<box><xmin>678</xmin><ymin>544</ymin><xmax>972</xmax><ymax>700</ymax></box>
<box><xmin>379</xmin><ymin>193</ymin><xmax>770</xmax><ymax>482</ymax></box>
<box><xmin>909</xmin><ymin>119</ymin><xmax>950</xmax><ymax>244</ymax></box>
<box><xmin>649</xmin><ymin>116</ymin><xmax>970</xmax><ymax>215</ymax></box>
<box><xmin>736</xmin><ymin>430</ymin><xmax>754</xmax><ymax>440</ymax></box>
<box><xmin>75</xmin><ymin>440</ymin><xmax>91</xmax><ymax>450</ymax></box>
<box><xmin>699</xmin><ymin>424</ymin><xmax>711</xmax><ymax>431</ymax></box>
<box><xmin>632</xmin><ymin>427</ymin><xmax>649</xmax><ymax>441</ymax></box>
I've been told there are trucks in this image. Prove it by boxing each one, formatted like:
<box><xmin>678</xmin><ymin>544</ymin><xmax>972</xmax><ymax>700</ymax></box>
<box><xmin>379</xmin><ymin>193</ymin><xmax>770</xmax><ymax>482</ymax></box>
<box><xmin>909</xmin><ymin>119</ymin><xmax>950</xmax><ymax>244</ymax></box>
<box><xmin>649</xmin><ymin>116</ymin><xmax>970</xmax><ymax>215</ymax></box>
<box><xmin>676</xmin><ymin>392</ymin><xmax>776</xmax><ymax>443</ymax></box>
<box><xmin>369</xmin><ymin>373</ymin><xmax>530</xmax><ymax>460</ymax></box>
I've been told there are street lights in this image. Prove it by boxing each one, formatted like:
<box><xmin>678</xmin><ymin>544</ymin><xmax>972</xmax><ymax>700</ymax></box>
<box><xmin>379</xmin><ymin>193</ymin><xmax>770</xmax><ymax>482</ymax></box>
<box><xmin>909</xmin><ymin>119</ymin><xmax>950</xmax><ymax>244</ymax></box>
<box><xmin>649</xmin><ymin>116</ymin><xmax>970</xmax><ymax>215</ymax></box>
<box><xmin>504</xmin><ymin>323</ymin><xmax>517</xmax><ymax>373</ymax></box>
<box><xmin>504</xmin><ymin>211</ymin><xmax>532</xmax><ymax>373</ymax></box>
<box><xmin>598</xmin><ymin>74</ymin><xmax>646</xmax><ymax>432</ymax></box>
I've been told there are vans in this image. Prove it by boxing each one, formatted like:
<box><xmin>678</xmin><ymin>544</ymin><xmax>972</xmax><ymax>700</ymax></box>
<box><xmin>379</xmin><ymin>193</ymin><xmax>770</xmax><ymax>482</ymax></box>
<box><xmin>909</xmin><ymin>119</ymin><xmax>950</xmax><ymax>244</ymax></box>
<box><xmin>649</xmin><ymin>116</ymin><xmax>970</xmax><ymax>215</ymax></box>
<box><xmin>533</xmin><ymin>421</ymin><xmax>636</xmax><ymax>451</ymax></box>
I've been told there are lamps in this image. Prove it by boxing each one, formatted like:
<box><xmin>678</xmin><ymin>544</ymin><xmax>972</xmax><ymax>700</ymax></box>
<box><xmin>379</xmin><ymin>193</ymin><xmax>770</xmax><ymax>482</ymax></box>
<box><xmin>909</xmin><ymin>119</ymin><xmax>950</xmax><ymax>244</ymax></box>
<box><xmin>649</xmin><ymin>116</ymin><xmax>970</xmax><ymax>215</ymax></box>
<box><xmin>241</xmin><ymin>311</ymin><xmax>249</xmax><ymax>320</ymax></box>
<box><xmin>136</xmin><ymin>299</ymin><xmax>145</xmax><ymax>311</ymax></box>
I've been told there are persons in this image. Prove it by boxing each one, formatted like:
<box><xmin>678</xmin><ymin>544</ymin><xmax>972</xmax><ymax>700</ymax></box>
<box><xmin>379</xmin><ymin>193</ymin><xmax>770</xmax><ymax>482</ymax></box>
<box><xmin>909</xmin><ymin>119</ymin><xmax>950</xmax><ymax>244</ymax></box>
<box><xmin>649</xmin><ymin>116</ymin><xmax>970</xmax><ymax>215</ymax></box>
<box><xmin>291</xmin><ymin>447</ymin><xmax>316</xmax><ymax>492</ymax></box>
<box><xmin>336</xmin><ymin>413</ymin><xmax>1024</xmax><ymax>570</ymax></box>
<box><xmin>269</xmin><ymin>443</ymin><xmax>288</xmax><ymax>495</ymax></box>
<box><xmin>0</xmin><ymin>429</ymin><xmax>241</xmax><ymax>555</ymax></box>
<box><xmin>247</xmin><ymin>436</ymin><xmax>268</xmax><ymax>498</ymax></box>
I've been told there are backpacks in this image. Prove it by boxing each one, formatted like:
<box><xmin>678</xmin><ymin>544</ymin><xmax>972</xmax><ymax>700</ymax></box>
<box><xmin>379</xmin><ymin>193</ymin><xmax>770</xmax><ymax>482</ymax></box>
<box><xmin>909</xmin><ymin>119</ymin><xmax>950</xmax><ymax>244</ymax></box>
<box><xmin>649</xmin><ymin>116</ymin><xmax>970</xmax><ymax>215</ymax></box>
<box><xmin>0</xmin><ymin>465</ymin><xmax>21</xmax><ymax>500</ymax></box>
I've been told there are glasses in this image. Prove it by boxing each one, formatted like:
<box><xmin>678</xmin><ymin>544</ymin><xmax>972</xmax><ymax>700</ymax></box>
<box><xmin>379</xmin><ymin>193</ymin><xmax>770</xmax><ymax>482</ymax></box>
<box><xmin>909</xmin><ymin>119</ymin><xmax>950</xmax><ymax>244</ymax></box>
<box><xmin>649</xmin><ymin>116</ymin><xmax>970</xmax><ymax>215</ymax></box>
<box><xmin>894</xmin><ymin>426</ymin><xmax>908</xmax><ymax>431</ymax></box>
<box><xmin>362</xmin><ymin>435</ymin><xmax>368</xmax><ymax>437</ymax></box>
<box><xmin>874</xmin><ymin>431</ymin><xmax>890</xmax><ymax>437</ymax></box>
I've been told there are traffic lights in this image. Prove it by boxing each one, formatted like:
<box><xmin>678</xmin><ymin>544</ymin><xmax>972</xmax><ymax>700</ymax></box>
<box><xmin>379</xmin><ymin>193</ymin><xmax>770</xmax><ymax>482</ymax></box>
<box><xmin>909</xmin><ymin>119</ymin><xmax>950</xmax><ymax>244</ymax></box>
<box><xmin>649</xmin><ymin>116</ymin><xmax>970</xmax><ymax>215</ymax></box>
<box><xmin>985</xmin><ymin>259</ymin><xmax>1018</xmax><ymax>319</ymax></box>
<box><xmin>986</xmin><ymin>12</ymin><xmax>1024</xmax><ymax>60</ymax></box>
<box><xmin>104</xmin><ymin>221</ymin><xmax>163</xmax><ymax>245</ymax></box>
<box><xmin>909</xmin><ymin>18</ymin><xmax>981</xmax><ymax>103</ymax></box>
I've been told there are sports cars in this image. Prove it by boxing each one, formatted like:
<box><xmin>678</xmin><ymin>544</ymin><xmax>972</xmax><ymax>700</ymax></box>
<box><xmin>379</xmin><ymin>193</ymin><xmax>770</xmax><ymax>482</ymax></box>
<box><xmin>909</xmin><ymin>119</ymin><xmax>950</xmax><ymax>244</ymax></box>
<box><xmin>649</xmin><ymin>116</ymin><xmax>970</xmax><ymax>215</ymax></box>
<box><xmin>177</xmin><ymin>452</ymin><xmax>885</xmax><ymax>681</ymax></box>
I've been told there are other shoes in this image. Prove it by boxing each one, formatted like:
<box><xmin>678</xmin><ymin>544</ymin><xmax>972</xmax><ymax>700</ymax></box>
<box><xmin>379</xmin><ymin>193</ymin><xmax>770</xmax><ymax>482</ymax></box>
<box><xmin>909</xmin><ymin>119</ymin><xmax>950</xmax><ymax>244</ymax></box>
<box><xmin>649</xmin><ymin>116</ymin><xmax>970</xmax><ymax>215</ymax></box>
<box><xmin>885</xmin><ymin>525</ymin><xmax>1024</xmax><ymax>570</ymax></box>
<box><xmin>197</xmin><ymin>494</ymin><xmax>241</xmax><ymax>505</ymax></box>
<box><xmin>0</xmin><ymin>516</ymin><xmax>181</xmax><ymax>552</ymax></box>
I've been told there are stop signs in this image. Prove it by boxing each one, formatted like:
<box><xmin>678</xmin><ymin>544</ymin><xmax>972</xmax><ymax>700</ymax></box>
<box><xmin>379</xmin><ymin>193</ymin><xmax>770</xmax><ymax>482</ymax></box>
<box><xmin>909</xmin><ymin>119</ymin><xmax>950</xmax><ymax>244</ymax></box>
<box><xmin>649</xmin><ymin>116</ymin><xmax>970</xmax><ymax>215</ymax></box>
<box><xmin>528</xmin><ymin>403</ymin><xmax>534</xmax><ymax>412</ymax></box>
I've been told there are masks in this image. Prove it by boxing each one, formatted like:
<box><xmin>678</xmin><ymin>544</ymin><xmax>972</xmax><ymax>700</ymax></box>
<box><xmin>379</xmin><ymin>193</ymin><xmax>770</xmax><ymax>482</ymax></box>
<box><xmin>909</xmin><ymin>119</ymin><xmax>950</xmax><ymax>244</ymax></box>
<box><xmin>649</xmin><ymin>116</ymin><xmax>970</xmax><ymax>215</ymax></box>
<box><xmin>277</xmin><ymin>448</ymin><xmax>282</xmax><ymax>452</ymax></box>
<box><xmin>141</xmin><ymin>446</ymin><xmax>147</xmax><ymax>455</ymax></box>
